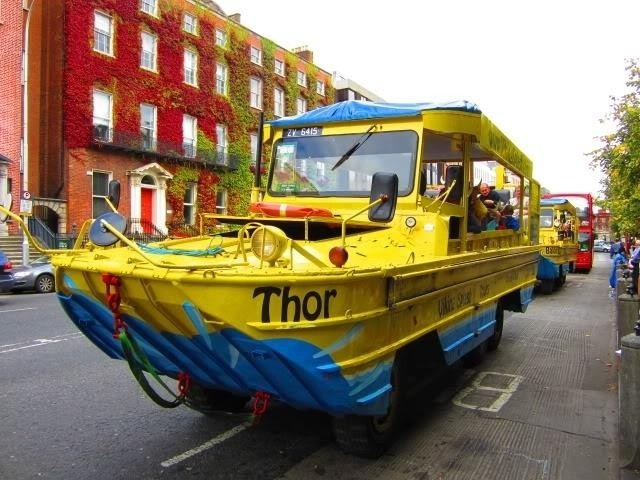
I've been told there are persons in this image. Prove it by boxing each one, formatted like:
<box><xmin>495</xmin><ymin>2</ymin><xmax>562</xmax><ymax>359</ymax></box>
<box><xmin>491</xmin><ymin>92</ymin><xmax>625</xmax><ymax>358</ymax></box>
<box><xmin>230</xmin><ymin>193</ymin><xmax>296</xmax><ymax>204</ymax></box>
<box><xmin>479</xmin><ymin>183</ymin><xmax>507</xmax><ymax>225</ymax></box>
<box><xmin>469</xmin><ymin>181</ymin><xmax>489</xmax><ymax>234</ymax></box>
<box><xmin>496</xmin><ymin>203</ymin><xmax>520</xmax><ymax>231</ymax></box>
<box><xmin>611</xmin><ymin>238</ymin><xmax>625</xmax><ymax>260</ymax></box>
<box><xmin>627</xmin><ymin>239</ymin><xmax>640</xmax><ymax>270</ymax></box>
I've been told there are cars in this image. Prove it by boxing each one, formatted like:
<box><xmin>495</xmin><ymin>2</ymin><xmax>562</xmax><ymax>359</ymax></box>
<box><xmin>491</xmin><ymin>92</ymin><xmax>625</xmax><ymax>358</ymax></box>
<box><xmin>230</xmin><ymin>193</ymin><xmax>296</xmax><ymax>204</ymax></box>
<box><xmin>594</xmin><ymin>242</ymin><xmax>611</xmax><ymax>253</ymax></box>
<box><xmin>0</xmin><ymin>251</ymin><xmax>15</xmax><ymax>293</ymax></box>
<box><xmin>11</xmin><ymin>255</ymin><xmax>54</xmax><ymax>293</ymax></box>
